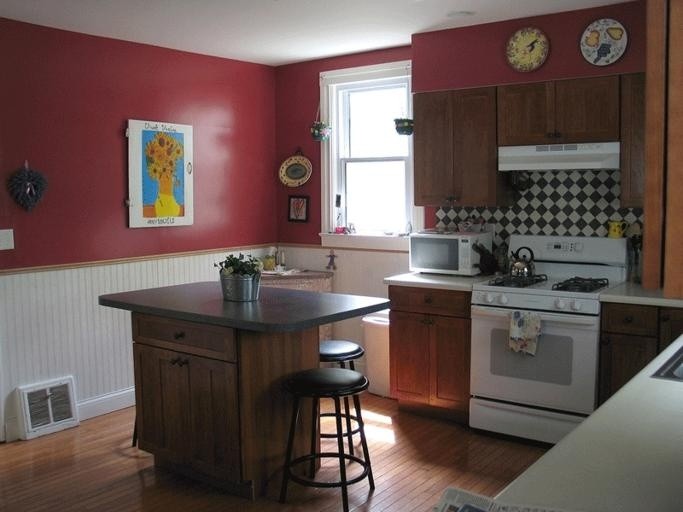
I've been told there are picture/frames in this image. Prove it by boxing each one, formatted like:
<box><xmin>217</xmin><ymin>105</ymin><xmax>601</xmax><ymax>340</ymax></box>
<box><xmin>285</xmin><ymin>193</ymin><xmax>309</xmax><ymax>223</ymax></box>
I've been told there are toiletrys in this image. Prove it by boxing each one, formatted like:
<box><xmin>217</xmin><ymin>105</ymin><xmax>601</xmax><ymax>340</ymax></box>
<box><xmin>263</xmin><ymin>246</ymin><xmax>277</xmax><ymax>271</ymax></box>
<box><xmin>280</xmin><ymin>250</ymin><xmax>287</xmax><ymax>266</ymax></box>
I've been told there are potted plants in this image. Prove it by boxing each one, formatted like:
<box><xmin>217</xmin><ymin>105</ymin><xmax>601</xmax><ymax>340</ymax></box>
<box><xmin>394</xmin><ymin>118</ymin><xmax>413</xmax><ymax>135</ymax></box>
<box><xmin>310</xmin><ymin>80</ymin><xmax>333</xmax><ymax>141</ymax></box>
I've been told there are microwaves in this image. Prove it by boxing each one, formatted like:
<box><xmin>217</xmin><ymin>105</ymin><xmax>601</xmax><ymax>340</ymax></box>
<box><xmin>410</xmin><ymin>231</ymin><xmax>494</xmax><ymax>277</ymax></box>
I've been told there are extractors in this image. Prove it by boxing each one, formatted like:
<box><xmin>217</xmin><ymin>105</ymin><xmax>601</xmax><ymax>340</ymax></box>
<box><xmin>497</xmin><ymin>143</ymin><xmax>621</xmax><ymax>172</ymax></box>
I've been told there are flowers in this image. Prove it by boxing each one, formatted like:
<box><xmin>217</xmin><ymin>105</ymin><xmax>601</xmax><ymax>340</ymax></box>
<box><xmin>206</xmin><ymin>252</ymin><xmax>263</xmax><ymax>282</ymax></box>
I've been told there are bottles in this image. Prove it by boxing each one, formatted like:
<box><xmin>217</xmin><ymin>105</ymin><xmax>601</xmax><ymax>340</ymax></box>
<box><xmin>259</xmin><ymin>245</ymin><xmax>286</xmax><ymax>269</ymax></box>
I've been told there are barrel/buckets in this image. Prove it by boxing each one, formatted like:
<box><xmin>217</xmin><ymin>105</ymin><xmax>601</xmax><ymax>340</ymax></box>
<box><xmin>220</xmin><ymin>273</ymin><xmax>260</xmax><ymax>301</ymax></box>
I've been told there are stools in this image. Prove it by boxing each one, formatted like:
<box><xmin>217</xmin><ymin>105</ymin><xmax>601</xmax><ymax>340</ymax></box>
<box><xmin>311</xmin><ymin>338</ymin><xmax>366</xmax><ymax>458</ymax></box>
<box><xmin>271</xmin><ymin>366</ymin><xmax>376</xmax><ymax>512</ymax></box>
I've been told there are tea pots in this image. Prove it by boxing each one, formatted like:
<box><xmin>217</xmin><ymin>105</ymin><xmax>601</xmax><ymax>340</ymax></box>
<box><xmin>607</xmin><ymin>220</ymin><xmax>628</xmax><ymax>239</ymax></box>
<box><xmin>509</xmin><ymin>248</ymin><xmax>538</xmax><ymax>280</ymax></box>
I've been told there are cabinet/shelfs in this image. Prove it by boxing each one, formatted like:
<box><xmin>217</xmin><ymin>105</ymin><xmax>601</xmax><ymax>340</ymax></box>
<box><xmin>620</xmin><ymin>69</ymin><xmax>646</xmax><ymax>207</ymax></box>
<box><xmin>410</xmin><ymin>84</ymin><xmax>514</xmax><ymax>208</ymax></box>
<box><xmin>496</xmin><ymin>73</ymin><xmax>621</xmax><ymax>146</ymax></box>
<box><xmin>596</xmin><ymin>297</ymin><xmax>656</xmax><ymax>413</ymax></box>
<box><xmin>383</xmin><ymin>280</ymin><xmax>470</xmax><ymax>428</ymax></box>
<box><xmin>657</xmin><ymin>306</ymin><xmax>682</xmax><ymax>353</ymax></box>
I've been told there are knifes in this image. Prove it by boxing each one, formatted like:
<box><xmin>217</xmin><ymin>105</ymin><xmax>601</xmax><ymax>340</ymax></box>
<box><xmin>470</xmin><ymin>242</ymin><xmax>487</xmax><ymax>269</ymax></box>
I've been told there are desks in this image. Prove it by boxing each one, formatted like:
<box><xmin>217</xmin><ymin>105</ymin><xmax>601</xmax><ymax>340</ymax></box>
<box><xmin>92</xmin><ymin>276</ymin><xmax>393</xmax><ymax>503</ymax></box>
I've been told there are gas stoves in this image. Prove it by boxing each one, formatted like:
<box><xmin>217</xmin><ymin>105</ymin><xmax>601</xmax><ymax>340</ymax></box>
<box><xmin>471</xmin><ymin>271</ymin><xmax>631</xmax><ymax>316</ymax></box>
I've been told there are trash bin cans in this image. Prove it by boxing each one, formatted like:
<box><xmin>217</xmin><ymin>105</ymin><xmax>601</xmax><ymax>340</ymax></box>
<box><xmin>362</xmin><ymin>308</ymin><xmax>396</xmax><ymax>400</ymax></box>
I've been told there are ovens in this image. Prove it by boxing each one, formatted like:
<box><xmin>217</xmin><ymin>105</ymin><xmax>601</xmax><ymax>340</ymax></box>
<box><xmin>471</xmin><ymin>303</ymin><xmax>601</xmax><ymax>450</ymax></box>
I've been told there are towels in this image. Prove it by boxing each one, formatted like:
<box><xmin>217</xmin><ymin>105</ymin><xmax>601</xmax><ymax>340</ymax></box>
<box><xmin>506</xmin><ymin>308</ymin><xmax>543</xmax><ymax>358</ymax></box>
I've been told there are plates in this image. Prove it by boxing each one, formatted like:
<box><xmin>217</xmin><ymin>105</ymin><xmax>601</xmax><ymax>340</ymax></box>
<box><xmin>579</xmin><ymin>16</ymin><xmax>627</xmax><ymax>68</ymax></box>
<box><xmin>504</xmin><ymin>28</ymin><xmax>549</xmax><ymax>74</ymax></box>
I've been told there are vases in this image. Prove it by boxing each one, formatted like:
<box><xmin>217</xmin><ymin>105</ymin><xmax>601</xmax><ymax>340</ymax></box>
<box><xmin>213</xmin><ymin>273</ymin><xmax>263</xmax><ymax>302</ymax></box>
